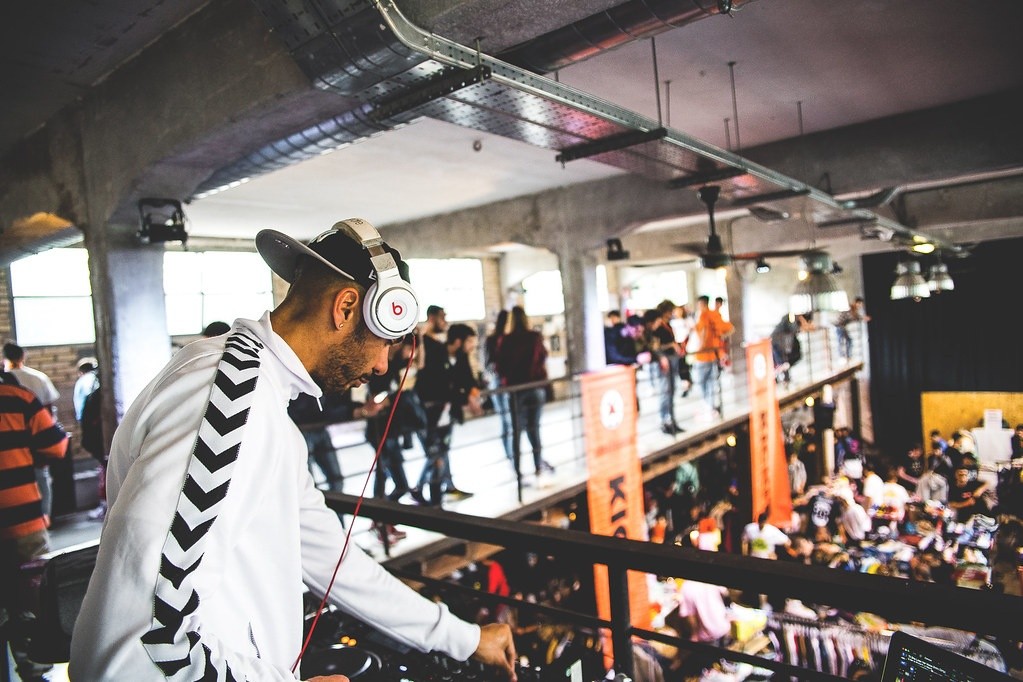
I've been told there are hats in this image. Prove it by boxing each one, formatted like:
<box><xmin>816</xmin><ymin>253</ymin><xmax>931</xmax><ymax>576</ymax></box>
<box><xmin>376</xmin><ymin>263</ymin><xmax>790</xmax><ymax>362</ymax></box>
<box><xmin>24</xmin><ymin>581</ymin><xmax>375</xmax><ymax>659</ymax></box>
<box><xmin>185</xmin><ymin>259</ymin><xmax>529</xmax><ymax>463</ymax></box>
<box><xmin>254</xmin><ymin>226</ymin><xmax>410</xmax><ymax>291</ymax></box>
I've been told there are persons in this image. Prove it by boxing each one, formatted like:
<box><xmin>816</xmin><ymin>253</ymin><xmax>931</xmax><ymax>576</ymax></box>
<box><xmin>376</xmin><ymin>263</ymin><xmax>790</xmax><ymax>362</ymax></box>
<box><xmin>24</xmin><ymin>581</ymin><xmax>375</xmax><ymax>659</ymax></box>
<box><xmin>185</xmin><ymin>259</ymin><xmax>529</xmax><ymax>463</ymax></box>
<box><xmin>837</xmin><ymin>297</ymin><xmax>870</xmax><ymax>362</ymax></box>
<box><xmin>0</xmin><ymin>341</ymin><xmax>107</xmax><ymax>681</ymax></box>
<box><xmin>70</xmin><ymin>218</ymin><xmax>516</xmax><ymax>681</ymax></box>
<box><xmin>479</xmin><ymin>428</ymin><xmax>1023</xmax><ymax>682</ymax></box>
<box><xmin>286</xmin><ymin>301</ymin><xmax>558</xmax><ymax>538</ymax></box>
<box><xmin>603</xmin><ymin>293</ymin><xmax>817</xmax><ymax>432</ymax></box>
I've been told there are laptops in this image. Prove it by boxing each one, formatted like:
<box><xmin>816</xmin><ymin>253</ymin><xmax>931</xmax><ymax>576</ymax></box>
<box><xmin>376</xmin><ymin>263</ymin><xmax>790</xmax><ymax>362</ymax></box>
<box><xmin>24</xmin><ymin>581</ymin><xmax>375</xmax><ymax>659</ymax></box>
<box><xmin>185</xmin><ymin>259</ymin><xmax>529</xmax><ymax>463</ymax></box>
<box><xmin>880</xmin><ymin>630</ymin><xmax>1023</xmax><ymax>682</ymax></box>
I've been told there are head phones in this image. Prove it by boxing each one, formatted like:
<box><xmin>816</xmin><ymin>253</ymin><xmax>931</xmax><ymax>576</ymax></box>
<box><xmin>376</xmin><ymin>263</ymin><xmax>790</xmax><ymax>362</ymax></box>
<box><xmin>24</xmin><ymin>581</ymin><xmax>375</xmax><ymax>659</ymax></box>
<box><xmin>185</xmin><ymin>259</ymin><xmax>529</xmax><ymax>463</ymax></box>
<box><xmin>331</xmin><ymin>218</ymin><xmax>420</xmax><ymax>340</ymax></box>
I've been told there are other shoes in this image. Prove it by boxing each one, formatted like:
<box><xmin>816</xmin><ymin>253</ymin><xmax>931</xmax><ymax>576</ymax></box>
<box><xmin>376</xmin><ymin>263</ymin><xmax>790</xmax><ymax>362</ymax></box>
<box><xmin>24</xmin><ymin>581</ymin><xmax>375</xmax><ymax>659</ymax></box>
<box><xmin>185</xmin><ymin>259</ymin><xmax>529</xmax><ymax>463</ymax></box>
<box><xmin>506</xmin><ymin>369</ymin><xmax>793</xmax><ymax>486</ymax></box>
<box><xmin>369</xmin><ymin>522</ymin><xmax>407</xmax><ymax>545</ymax></box>
<box><xmin>411</xmin><ymin>486</ymin><xmax>426</xmax><ymax>504</ymax></box>
<box><xmin>443</xmin><ymin>489</ymin><xmax>473</xmax><ymax>500</ymax></box>
